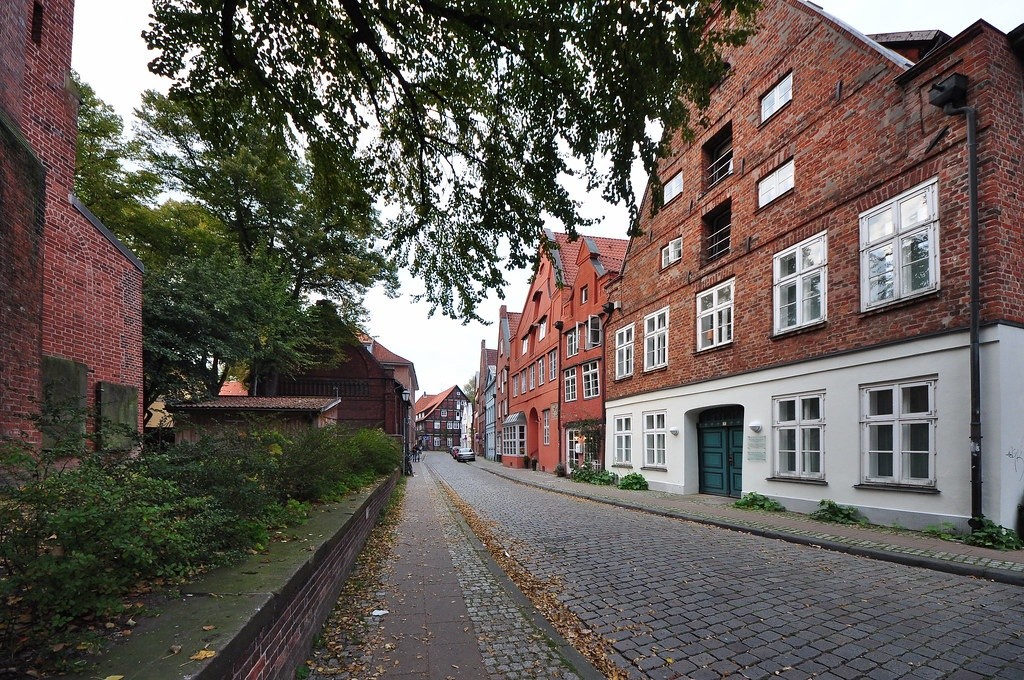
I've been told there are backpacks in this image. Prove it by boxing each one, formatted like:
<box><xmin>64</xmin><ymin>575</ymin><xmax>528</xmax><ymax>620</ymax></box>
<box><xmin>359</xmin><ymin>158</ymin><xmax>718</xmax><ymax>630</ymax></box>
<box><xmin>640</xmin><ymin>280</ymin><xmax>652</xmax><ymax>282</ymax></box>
<box><xmin>412</xmin><ymin>447</ymin><xmax>417</xmax><ymax>452</ymax></box>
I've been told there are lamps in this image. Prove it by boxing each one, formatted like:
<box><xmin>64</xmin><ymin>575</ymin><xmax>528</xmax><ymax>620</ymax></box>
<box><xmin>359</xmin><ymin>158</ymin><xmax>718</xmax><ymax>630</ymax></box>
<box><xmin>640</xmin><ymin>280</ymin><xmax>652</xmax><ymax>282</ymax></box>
<box><xmin>748</xmin><ymin>421</ymin><xmax>761</xmax><ymax>432</ymax></box>
<box><xmin>669</xmin><ymin>427</ymin><xmax>679</xmax><ymax>436</ymax></box>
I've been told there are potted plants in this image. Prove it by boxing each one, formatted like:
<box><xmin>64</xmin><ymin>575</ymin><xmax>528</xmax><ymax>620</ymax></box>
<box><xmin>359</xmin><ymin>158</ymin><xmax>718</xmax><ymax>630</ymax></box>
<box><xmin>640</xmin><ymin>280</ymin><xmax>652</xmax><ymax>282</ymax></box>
<box><xmin>523</xmin><ymin>456</ymin><xmax>530</xmax><ymax>467</ymax></box>
<box><xmin>557</xmin><ymin>464</ymin><xmax>564</xmax><ymax>477</ymax></box>
<box><xmin>532</xmin><ymin>460</ymin><xmax>537</xmax><ymax>471</ymax></box>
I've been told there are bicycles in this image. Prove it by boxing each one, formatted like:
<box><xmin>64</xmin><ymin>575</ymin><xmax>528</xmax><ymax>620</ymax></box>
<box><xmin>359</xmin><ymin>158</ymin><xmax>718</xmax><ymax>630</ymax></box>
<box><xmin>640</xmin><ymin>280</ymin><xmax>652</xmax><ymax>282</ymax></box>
<box><xmin>416</xmin><ymin>451</ymin><xmax>422</xmax><ymax>462</ymax></box>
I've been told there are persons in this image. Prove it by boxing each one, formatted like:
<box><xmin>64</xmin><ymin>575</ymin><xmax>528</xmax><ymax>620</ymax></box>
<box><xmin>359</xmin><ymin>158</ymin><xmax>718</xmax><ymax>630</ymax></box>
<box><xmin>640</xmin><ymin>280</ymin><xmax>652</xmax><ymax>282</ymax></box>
<box><xmin>412</xmin><ymin>444</ymin><xmax>422</xmax><ymax>462</ymax></box>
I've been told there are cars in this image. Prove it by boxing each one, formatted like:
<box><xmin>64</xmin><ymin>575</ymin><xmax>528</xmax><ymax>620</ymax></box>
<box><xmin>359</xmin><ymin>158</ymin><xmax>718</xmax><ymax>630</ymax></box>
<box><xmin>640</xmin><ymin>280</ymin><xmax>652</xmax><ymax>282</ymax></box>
<box><xmin>457</xmin><ymin>447</ymin><xmax>475</xmax><ymax>462</ymax></box>
<box><xmin>448</xmin><ymin>445</ymin><xmax>463</xmax><ymax>459</ymax></box>
